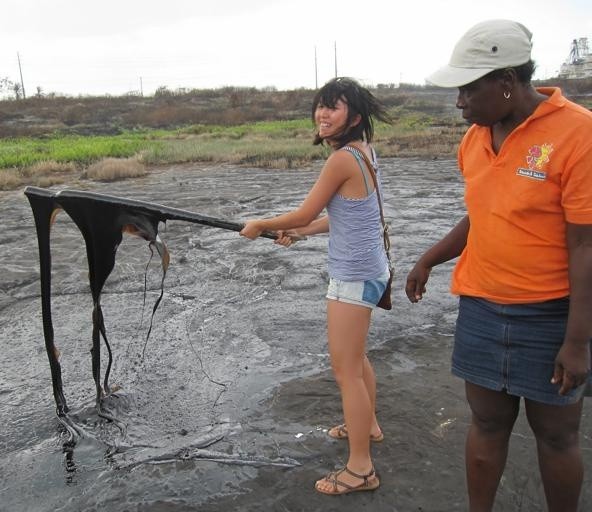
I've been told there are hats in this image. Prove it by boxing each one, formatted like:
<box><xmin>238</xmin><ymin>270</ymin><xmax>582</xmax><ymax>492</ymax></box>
<box><xmin>424</xmin><ymin>19</ymin><xmax>532</xmax><ymax>88</ymax></box>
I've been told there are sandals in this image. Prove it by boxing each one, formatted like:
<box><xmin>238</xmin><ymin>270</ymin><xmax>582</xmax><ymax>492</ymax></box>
<box><xmin>328</xmin><ymin>418</ymin><xmax>383</xmax><ymax>443</ymax></box>
<box><xmin>315</xmin><ymin>465</ymin><xmax>381</xmax><ymax>495</ymax></box>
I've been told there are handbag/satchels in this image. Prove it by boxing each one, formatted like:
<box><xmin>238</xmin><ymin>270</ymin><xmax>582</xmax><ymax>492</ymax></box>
<box><xmin>373</xmin><ymin>267</ymin><xmax>395</xmax><ymax>310</ymax></box>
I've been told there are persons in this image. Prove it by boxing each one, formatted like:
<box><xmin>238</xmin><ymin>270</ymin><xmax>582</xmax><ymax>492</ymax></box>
<box><xmin>402</xmin><ymin>20</ymin><xmax>591</xmax><ymax>511</ymax></box>
<box><xmin>239</xmin><ymin>78</ymin><xmax>394</xmax><ymax>494</ymax></box>
